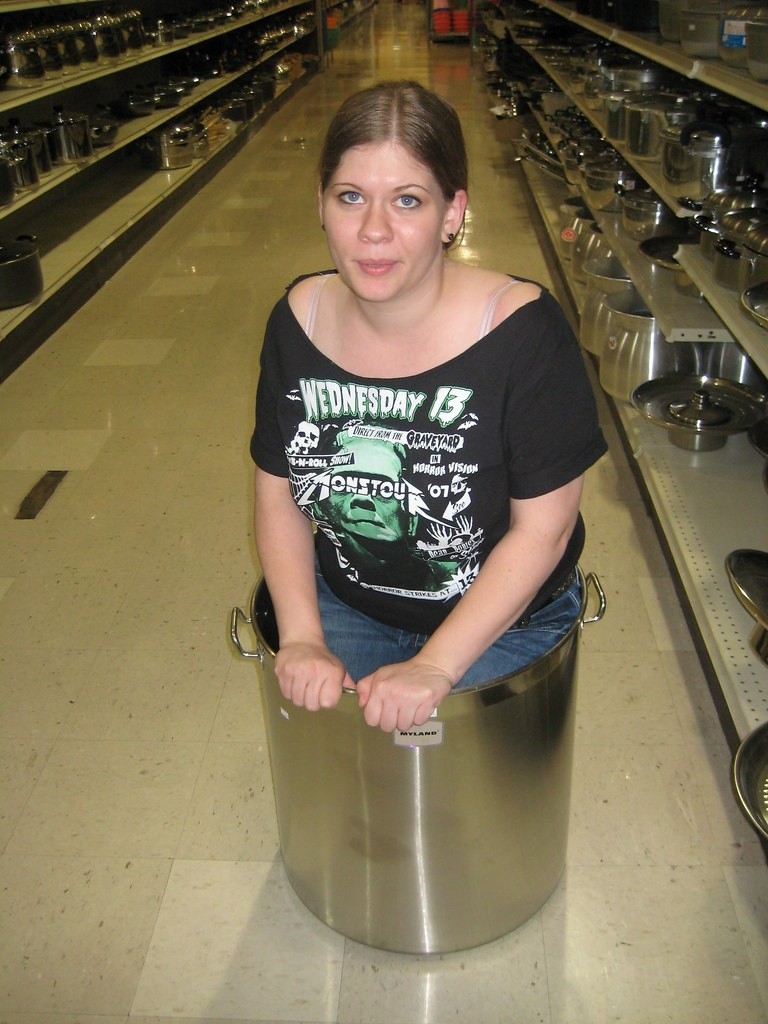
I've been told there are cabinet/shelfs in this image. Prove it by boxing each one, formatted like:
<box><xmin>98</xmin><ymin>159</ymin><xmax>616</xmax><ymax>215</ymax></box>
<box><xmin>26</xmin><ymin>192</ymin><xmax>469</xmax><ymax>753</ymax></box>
<box><xmin>0</xmin><ymin>0</ymin><xmax>323</xmax><ymax>341</ymax></box>
<box><xmin>476</xmin><ymin>0</ymin><xmax>768</xmax><ymax>820</ymax></box>
<box><xmin>322</xmin><ymin>0</ymin><xmax>375</xmax><ymax>30</ymax></box>
<box><xmin>426</xmin><ymin>0</ymin><xmax>475</xmax><ymax>48</ymax></box>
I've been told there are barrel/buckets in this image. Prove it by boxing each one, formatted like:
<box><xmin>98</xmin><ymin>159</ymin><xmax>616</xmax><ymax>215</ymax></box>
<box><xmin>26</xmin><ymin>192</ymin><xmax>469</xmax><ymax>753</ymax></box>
<box><xmin>226</xmin><ymin>564</ymin><xmax>607</xmax><ymax>954</ymax></box>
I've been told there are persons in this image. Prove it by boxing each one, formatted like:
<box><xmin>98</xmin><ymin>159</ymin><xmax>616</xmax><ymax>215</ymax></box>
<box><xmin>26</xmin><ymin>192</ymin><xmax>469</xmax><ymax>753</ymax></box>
<box><xmin>248</xmin><ymin>80</ymin><xmax>609</xmax><ymax>735</ymax></box>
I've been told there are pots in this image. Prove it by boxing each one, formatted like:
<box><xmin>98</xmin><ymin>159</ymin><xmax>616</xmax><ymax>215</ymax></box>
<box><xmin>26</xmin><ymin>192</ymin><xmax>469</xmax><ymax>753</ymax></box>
<box><xmin>1</xmin><ymin>1</ymin><xmax>316</xmax><ymax>311</ymax></box>
<box><xmin>474</xmin><ymin>1</ymin><xmax>768</xmax><ymax>398</ymax></box>
